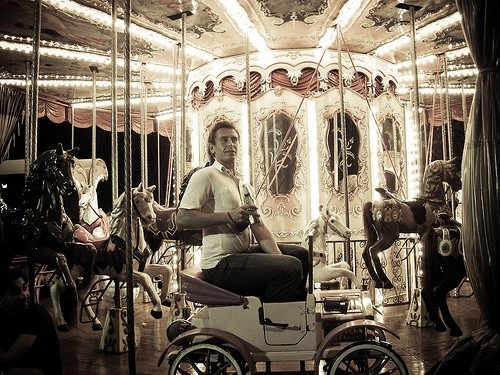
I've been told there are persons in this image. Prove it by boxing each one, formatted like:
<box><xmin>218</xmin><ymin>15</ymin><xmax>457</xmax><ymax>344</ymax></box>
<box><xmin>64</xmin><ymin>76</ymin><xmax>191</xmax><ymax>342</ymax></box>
<box><xmin>175</xmin><ymin>120</ymin><xmax>311</xmax><ymax>303</ymax></box>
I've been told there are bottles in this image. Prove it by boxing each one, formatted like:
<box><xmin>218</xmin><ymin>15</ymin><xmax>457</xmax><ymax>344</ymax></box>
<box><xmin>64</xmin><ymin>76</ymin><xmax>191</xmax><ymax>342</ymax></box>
<box><xmin>241</xmin><ymin>184</ymin><xmax>260</xmax><ymax>225</ymax></box>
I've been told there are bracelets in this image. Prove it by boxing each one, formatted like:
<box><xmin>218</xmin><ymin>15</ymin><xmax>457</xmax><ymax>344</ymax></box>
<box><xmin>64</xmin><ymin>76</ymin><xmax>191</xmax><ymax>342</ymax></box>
<box><xmin>228</xmin><ymin>211</ymin><xmax>238</xmax><ymax>225</ymax></box>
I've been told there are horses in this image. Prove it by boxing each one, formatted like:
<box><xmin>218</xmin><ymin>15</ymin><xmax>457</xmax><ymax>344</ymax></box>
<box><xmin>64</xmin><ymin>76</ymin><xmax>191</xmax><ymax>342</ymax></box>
<box><xmin>0</xmin><ymin>143</ymin><xmax>209</xmax><ymax>332</ymax></box>
<box><xmin>297</xmin><ymin>156</ymin><xmax>468</xmax><ymax>337</ymax></box>
